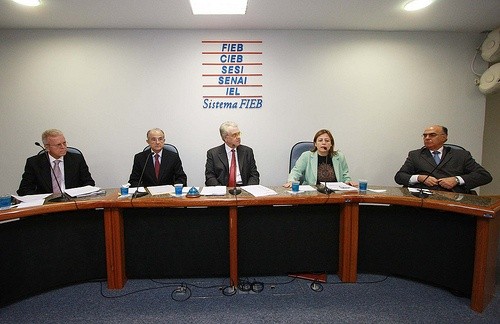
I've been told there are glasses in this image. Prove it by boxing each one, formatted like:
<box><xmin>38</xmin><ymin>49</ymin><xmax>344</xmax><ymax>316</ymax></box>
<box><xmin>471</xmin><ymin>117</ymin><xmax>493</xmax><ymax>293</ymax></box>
<box><xmin>421</xmin><ymin>134</ymin><xmax>445</xmax><ymax>140</ymax></box>
<box><xmin>46</xmin><ymin>143</ymin><xmax>67</xmax><ymax>148</ymax></box>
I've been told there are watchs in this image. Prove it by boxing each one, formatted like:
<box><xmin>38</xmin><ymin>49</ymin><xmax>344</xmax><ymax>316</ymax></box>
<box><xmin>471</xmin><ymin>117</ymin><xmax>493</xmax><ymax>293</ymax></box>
<box><xmin>455</xmin><ymin>177</ymin><xmax>460</xmax><ymax>183</ymax></box>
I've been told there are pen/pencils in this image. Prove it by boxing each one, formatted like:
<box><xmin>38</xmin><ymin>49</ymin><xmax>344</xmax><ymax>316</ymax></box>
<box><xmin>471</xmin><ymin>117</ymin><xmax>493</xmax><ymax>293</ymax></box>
<box><xmin>418</xmin><ymin>189</ymin><xmax>429</xmax><ymax>191</ymax></box>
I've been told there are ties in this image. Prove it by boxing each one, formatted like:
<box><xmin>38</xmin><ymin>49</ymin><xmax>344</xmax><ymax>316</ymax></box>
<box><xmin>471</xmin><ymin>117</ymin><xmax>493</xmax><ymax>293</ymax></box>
<box><xmin>228</xmin><ymin>149</ymin><xmax>236</xmax><ymax>187</ymax></box>
<box><xmin>432</xmin><ymin>150</ymin><xmax>441</xmax><ymax>165</ymax></box>
<box><xmin>52</xmin><ymin>160</ymin><xmax>62</xmax><ymax>193</ymax></box>
<box><xmin>154</xmin><ymin>153</ymin><xmax>161</xmax><ymax>179</ymax></box>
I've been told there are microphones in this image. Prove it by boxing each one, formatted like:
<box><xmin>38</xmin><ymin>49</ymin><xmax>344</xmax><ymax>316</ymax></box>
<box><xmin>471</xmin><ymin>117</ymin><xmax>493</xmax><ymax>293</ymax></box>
<box><xmin>411</xmin><ymin>147</ymin><xmax>451</xmax><ymax>199</ymax></box>
<box><xmin>229</xmin><ymin>150</ymin><xmax>241</xmax><ymax>196</ymax></box>
<box><xmin>132</xmin><ymin>152</ymin><xmax>155</xmax><ymax>198</ymax></box>
<box><xmin>317</xmin><ymin>147</ymin><xmax>332</xmax><ymax>193</ymax></box>
<box><xmin>35</xmin><ymin>142</ymin><xmax>70</xmax><ymax>202</ymax></box>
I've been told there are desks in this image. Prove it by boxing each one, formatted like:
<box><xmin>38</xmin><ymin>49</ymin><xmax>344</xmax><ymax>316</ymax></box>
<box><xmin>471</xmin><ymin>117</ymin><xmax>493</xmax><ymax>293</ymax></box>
<box><xmin>0</xmin><ymin>185</ymin><xmax>500</xmax><ymax>312</ymax></box>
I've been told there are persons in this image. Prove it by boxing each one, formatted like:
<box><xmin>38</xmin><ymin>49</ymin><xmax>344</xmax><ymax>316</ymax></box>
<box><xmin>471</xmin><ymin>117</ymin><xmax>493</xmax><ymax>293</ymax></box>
<box><xmin>282</xmin><ymin>129</ymin><xmax>359</xmax><ymax>188</ymax></box>
<box><xmin>206</xmin><ymin>121</ymin><xmax>260</xmax><ymax>186</ymax></box>
<box><xmin>394</xmin><ymin>125</ymin><xmax>494</xmax><ymax>195</ymax></box>
<box><xmin>16</xmin><ymin>130</ymin><xmax>96</xmax><ymax>196</ymax></box>
<box><xmin>128</xmin><ymin>128</ymin><xmax>188</xmax><ymax>186</ymax></box>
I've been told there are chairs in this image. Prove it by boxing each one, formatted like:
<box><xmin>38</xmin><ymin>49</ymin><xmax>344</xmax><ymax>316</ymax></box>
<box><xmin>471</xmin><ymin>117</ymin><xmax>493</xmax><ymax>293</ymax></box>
<box><xmin>289</xmin><ymin>142</ymin><xmax>316</xmax><ymax>181</ymax></box>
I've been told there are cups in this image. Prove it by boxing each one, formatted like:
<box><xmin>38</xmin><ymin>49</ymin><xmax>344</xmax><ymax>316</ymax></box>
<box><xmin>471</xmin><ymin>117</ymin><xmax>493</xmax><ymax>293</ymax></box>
<box><xmin>291</xmin><ymin>181</ymin><xmax>300</xmax><ymax>192</ymax></box>
<box><xmin>174</xmin><ymin>184</ymin><xmax>184</xmax><ymax>194</ymax></box>
<box><xmin>358</xmin><ymin>180</ymin><xmax>368</xmax><ymax>191</ymax></box>
<box><xmin>120</xmin><ymin>185</ymin><xmax>128</xmax><ymax>195</ymax></box>
<box><xmin>0</xmin><ymin>193</ymin><xmax>11</xmax><ymax>208</ymax></box>
